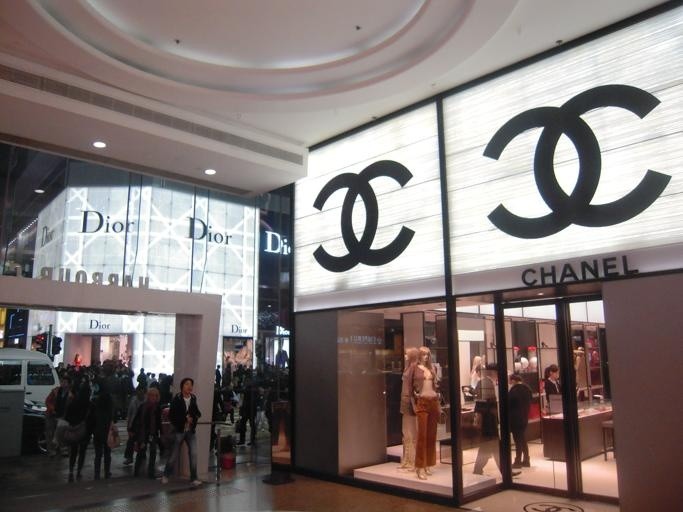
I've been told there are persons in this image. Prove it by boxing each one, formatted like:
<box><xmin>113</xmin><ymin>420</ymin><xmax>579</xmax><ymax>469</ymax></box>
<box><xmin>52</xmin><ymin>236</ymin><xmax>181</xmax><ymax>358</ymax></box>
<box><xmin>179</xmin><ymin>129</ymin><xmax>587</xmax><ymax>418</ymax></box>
<box><xmin>273</xmin><ymin>337</ymin><xmax>289</xmax><ymax>368</ymax></box>
<box><xmin>472</xmin><ymin>362</ymin><xmax>521</xmax><ymax>477</ymax></box>
<box><xmin>407</xmin><ymin>345</ymin><xmax>441</xmax><ymax>479</ymax></box>
<box><xmin>543</xmin><ymin>363</ymin><xmax>563</xmax><ymax>403</ymax></box>
<box><xmin>394</xmin><ymin>347</ymin><xmax>419</xmax><ymax>472</ymax></box>
<box><xmin>462</xmin><ymin>363</ymin><xmax>494</xmax><ymax>459</ymax></box>
<box><xmin>39</xmin><ymin>352</ymin><xmax>289</xmax><ymax>489</ymax></box>
<box><xmin>505</xmin><ymin>370</ymin><xmax>531</xmax><ymax>468</ymax></box>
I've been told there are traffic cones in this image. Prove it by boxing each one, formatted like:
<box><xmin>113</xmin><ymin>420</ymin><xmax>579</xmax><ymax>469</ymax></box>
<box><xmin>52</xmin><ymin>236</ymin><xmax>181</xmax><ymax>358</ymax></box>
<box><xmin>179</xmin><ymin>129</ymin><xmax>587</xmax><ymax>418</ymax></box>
<box><xmin>275</xmin><ymin>421</ymin><xmax>289</xmax><ymax>450</ymax></box>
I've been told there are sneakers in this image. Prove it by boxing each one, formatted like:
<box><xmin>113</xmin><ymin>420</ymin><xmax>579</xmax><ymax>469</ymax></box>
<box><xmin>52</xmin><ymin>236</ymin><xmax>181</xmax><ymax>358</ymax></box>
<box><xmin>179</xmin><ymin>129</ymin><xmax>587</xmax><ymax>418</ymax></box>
<box><xmin>160</xmin><ymin>476</ymin><xmax>168</xmax><ymax>485</ymax></box>
<box><xmin>190</xmin><ymin>480</ymin><xmax>202</xmax><ymax>486</ymax></box>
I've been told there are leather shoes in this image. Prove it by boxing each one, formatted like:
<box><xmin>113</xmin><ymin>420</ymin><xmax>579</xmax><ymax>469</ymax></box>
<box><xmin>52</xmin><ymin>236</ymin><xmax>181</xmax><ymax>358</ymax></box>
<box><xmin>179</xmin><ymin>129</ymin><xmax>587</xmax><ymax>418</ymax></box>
<box><xmin>511</xmin><ymin>471</ymin><xmax>520</xmax><ymax>477</ymax></box>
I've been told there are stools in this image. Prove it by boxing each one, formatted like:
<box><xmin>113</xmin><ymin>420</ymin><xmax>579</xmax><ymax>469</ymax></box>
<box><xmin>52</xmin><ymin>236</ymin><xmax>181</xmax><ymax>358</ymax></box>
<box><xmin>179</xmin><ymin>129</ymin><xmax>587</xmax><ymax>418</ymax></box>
<box><xmin>600</xmin><ymin>417</ymin><xmax>616</xmax><ymax>463</ymax></box>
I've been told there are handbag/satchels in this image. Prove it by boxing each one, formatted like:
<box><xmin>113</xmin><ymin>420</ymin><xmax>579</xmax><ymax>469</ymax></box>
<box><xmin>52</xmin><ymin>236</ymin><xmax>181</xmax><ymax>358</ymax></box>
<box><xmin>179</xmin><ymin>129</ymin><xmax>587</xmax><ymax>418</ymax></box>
<box><xmin>106</xmin><ymin>425</ymin><xmax>121</xmax><ymax>448</ymax></box>
<box><xmin>235</xmin><ymin>420</ymin><xmax>246</xmax><ymax>433</ymax></box>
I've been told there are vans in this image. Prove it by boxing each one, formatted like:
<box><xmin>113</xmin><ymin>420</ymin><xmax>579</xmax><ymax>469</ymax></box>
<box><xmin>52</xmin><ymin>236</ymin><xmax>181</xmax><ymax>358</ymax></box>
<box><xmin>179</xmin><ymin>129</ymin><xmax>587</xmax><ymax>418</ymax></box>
<box><xmin>0</xmin><ymin>347</ymin><xmax>60</xmax><ymax>404</ymax></box>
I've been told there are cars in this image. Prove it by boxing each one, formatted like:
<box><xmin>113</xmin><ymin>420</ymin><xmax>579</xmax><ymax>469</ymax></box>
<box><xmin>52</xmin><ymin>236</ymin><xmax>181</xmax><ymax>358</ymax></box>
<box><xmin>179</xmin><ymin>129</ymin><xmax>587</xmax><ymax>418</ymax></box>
<box><xmin>20</xmin><ymin>398</ymin><xmax>92</xmax><ymax>456</ymax></box>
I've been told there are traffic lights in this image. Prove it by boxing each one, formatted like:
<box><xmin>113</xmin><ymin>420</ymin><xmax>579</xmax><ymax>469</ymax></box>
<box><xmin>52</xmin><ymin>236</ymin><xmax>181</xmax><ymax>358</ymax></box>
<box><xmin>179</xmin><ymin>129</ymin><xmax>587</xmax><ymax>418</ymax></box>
<box><xmin>35</xmin><ymin>332</ymin><xmax>48</xmax><ymax>355</ymax></box>
<box><xmin>50</xmin><ymin>334</ymin><xmax>61</xmax><ymax>355</ymax></box>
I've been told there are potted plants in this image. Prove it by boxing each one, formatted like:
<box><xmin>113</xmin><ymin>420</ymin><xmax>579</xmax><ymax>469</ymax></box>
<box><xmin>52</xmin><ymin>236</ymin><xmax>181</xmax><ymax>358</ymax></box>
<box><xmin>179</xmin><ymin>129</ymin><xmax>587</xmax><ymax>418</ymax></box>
<box><xmin>213</xmin><ymin>432</ymin><xmax>239</xmax><ymax>472</ymax></box>
